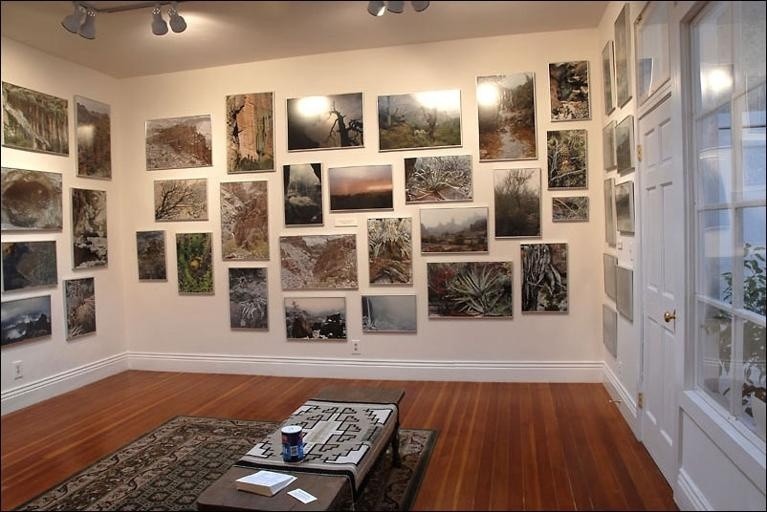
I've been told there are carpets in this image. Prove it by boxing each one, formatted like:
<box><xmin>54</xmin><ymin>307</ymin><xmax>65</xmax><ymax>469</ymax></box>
<box><xmin>9</xmin><ymin>414</ymin><xmax>441</xmax><ymax>512</ymax></box>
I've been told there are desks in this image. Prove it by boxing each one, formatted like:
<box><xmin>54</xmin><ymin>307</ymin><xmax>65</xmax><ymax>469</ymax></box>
<box><xmin>196</xmin><ymin>387</ymin><xmax>405</xmax><ymax>512</ymax></box>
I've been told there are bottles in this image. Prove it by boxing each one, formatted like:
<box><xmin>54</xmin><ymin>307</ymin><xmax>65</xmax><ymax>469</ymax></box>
<box><xmin>280</xmin><ymin>425</ymin><xmax>304</xmax><ymax>463</ymax></box>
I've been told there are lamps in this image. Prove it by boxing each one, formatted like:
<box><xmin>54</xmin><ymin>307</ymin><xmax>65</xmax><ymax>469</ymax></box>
<box><xmin>367</xmin><ymin>0</ymin><xmax>430</xmax><ymax>17</ymax></box>
<box><xmin>61</xmin><ymin>0</ymin><xmax>186</xmax><ymax>39</ymax></box>
